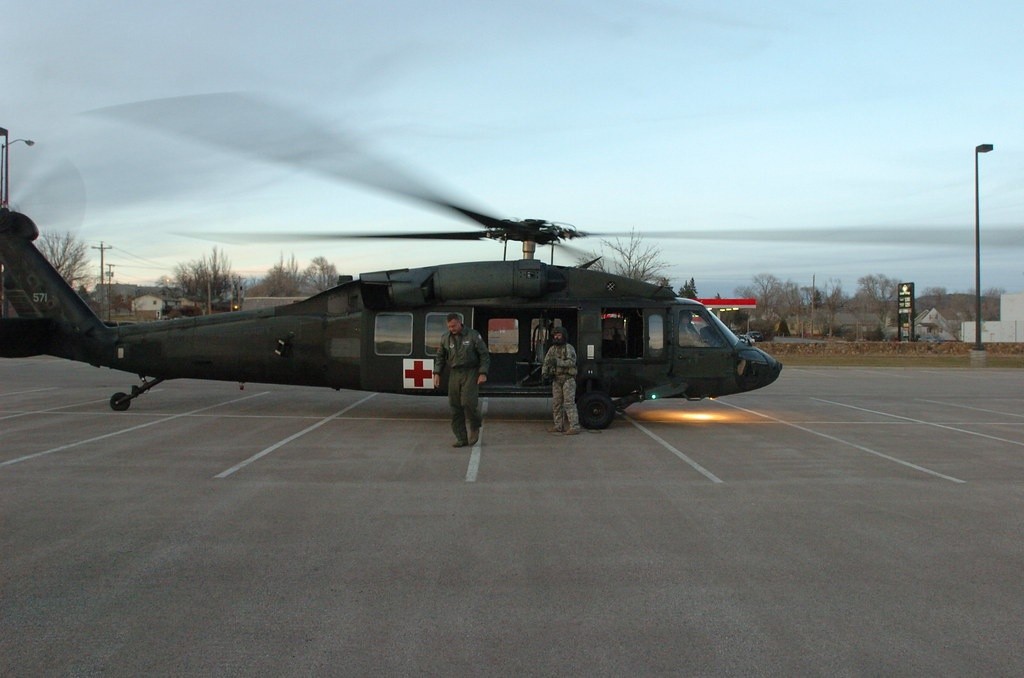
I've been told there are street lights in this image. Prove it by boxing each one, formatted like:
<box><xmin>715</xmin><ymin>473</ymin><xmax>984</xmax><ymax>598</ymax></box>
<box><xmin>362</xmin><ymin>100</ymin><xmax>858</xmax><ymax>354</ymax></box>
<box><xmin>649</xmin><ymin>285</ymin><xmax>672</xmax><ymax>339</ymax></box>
<box><xmin>975</xmin><ymin>143</ymin><xmax>994</xmax><ymax>350</ymax></box>
<box><xmin>1</xmin><ymin>130</ymin><xmax>35</xmax><ymax>208</ymax></box>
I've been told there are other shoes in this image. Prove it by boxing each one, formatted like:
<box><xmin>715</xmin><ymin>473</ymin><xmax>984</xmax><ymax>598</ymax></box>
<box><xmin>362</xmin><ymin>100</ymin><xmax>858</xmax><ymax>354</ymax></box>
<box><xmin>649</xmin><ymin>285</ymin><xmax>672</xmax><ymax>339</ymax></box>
<box><xmin>564</xmin><ymin>428</ymin><xmax>581</xmax><ymax>435</ymax></box>
<box><xmin>453</xmin><ymin>440</ymin><xmax>467</xmax><ymax>448</ymax></box>
<box><xmin>547</xmin><ymin>427</ymin><xmax>562</xmax><ymax>432</ymax></box>
<box><xmin>467</xmin><ymin>425</ymin><xmax>480</xmax><ymax>446</ymax></box>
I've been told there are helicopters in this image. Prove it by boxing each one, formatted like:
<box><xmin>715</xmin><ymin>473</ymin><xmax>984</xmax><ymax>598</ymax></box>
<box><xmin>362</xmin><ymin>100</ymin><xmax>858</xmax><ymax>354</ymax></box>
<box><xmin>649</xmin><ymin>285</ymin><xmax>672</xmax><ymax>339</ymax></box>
<box><xmin>1</xmin><ymin>94</ymin><xmax>1023</xmax><ymax>432</ymax></box>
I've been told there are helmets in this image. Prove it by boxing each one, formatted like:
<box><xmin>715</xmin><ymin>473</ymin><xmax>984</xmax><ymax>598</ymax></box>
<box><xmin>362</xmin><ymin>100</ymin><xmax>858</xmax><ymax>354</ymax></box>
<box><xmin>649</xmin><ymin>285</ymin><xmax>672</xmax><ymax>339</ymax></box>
<box><xmin>551</xmin><ymin>326</ymin><xmax>567</xmax><ymax>341</ymax></box>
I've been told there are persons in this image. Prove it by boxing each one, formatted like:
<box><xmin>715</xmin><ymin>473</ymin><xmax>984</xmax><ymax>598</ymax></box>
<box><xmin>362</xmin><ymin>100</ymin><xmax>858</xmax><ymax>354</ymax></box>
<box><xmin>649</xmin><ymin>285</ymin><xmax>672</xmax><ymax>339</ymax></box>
<box><xmin>679</xmin><ymin>311</ymin><xmax>710</xmax><ymax>347</ymax></box>
<box><xmin>433</xmin><ymin>312</ymin><xmax>491</xmax><ymax>448</ymax></box>
<box><xmin>542</xmin><ymin>326</ymin><xmax>581</xmax><ymax>435</ymax></box>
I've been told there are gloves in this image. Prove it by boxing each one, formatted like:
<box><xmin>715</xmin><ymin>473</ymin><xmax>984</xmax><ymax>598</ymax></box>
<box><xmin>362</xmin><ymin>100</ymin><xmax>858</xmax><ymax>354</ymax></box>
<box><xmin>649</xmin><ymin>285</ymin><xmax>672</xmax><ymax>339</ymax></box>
<box><xmin>551</xmin><ymin>359</ymin><xmax>557</xmax><ymax>366</ymax></box>
<box><xmin>542</xmin><ymin>374</ymin><xmax>549</xmax><ymax>385</ymax></box>
